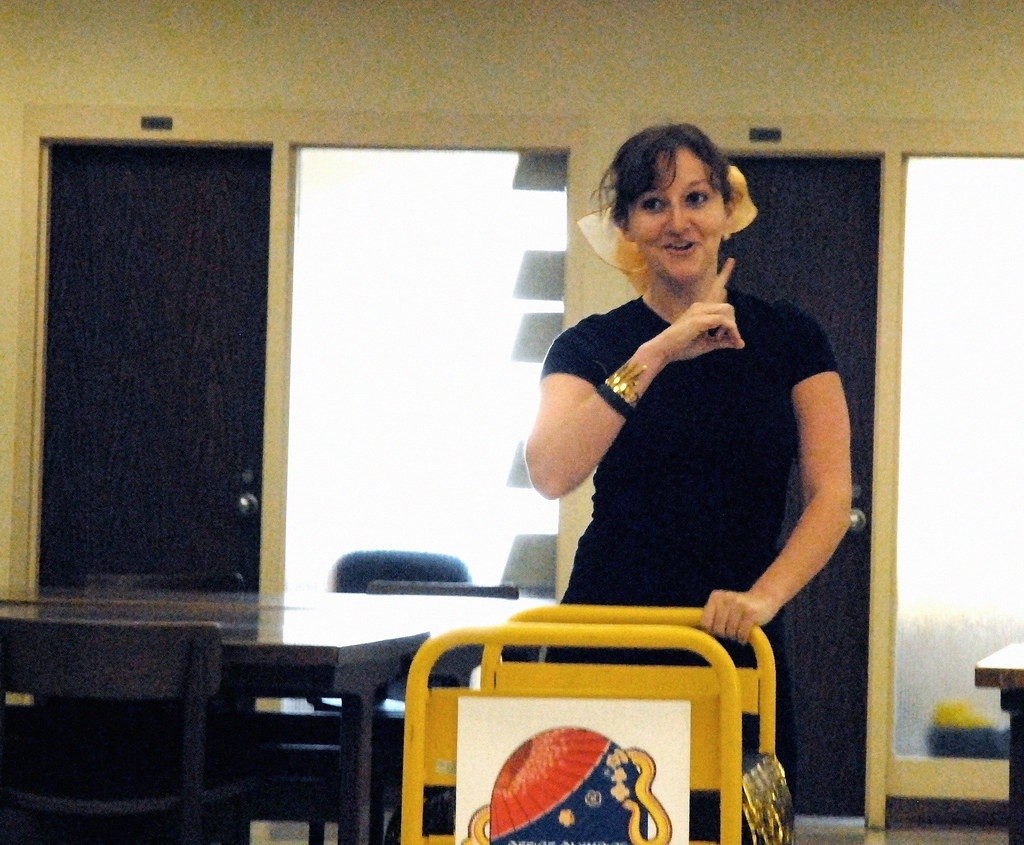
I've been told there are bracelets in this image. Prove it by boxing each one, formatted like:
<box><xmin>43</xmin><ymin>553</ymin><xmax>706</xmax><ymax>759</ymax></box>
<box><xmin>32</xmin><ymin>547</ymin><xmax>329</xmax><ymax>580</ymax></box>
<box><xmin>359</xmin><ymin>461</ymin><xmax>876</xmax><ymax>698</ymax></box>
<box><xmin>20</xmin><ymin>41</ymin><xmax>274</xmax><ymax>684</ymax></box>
<box><xmin>593</xmin><ymin>362</ymin><xmax>646</xmax><ymax>420</ymax></box>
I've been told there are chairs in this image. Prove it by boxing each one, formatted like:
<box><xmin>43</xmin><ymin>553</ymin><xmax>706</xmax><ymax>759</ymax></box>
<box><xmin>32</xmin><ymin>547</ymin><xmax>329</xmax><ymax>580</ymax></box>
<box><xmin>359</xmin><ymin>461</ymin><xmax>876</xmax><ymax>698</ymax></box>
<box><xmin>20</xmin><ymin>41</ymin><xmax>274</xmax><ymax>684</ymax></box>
<box><xmin>1</xmin><ymin>615</ymin><xmax>252</xmax><ymax>845</ymax></box>
<box><xmin>267</xmin><ymin>551</ymin><xmax>472</xmax><ymax>845</ymax></box>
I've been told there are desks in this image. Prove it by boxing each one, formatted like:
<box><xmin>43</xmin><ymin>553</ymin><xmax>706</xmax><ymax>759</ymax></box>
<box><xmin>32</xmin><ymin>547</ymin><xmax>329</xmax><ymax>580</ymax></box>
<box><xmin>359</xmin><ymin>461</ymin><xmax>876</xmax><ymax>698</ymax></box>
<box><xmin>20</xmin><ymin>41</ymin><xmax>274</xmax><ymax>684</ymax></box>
<box><xmin>0</xmin><ymin>588</ymin><xmax>555</xmax><ymax>845</ymax></box>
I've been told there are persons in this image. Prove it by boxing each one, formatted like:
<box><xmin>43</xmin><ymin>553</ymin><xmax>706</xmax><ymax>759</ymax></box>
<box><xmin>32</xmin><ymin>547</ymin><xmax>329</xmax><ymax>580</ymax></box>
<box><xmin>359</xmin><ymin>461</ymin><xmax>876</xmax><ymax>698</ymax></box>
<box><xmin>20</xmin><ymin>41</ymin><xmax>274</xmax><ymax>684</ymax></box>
<box><xmin>526</xmin><ymin>122</ymin><xmax>854</xmax><ymax>845</ymax></box>
